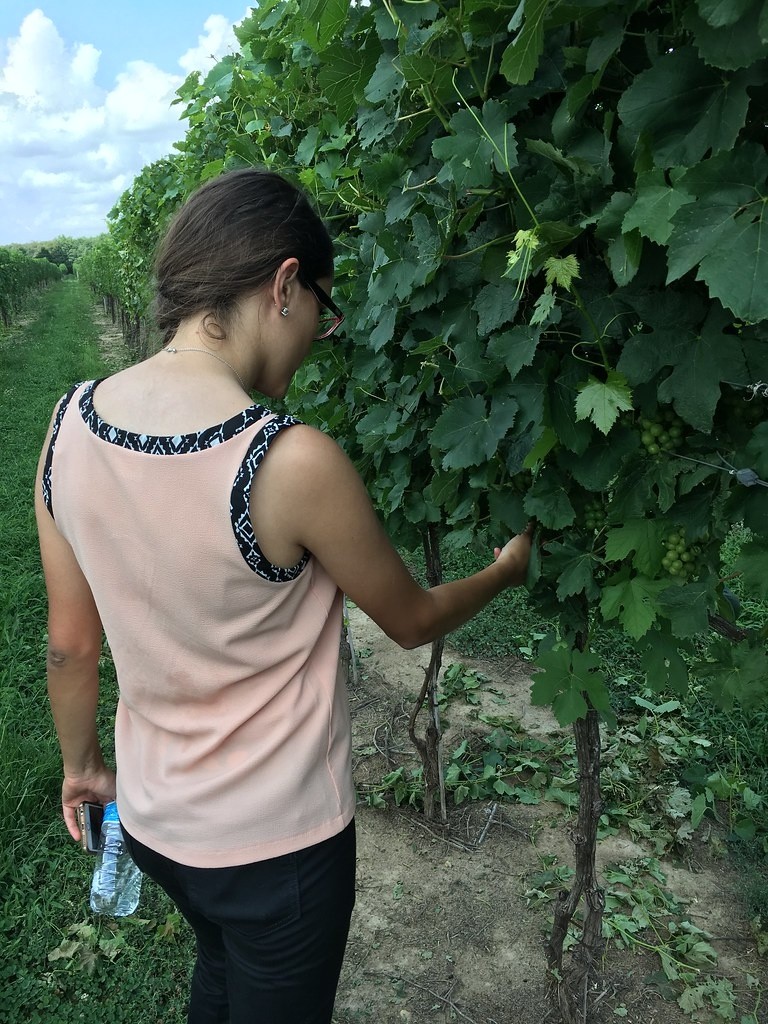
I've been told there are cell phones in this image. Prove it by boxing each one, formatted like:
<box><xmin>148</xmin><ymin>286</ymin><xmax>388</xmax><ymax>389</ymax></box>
<box><xmin>78</xmin><ymin>801</ymin><xmax>104</xmax><ymax>855</ymax></box>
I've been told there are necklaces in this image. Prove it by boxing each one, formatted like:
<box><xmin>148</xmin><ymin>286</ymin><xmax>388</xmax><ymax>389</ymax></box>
<box><xmin>161</xmin><ymin>347</ymin><xmax>247</xmax><ymax>392</ymax></box>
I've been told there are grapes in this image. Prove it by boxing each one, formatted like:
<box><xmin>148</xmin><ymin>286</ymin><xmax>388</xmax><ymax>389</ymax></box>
<box><xmin>579</xmin><ymin>406</ymin><xmax>706</xmax><ymax>580</ymax></box>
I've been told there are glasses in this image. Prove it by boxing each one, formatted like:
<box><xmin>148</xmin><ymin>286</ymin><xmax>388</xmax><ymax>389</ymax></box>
<box><xmin>284</xmin><ymin>254</ymin><xmax>346</xmax><ymax>342</ymax></box>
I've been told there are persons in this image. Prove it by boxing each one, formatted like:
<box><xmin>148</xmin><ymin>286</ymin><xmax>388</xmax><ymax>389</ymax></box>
<box><xmin>31</xmin><ymin>168</ymin><xmax>535</xmax><ymax>1023</ymax></box>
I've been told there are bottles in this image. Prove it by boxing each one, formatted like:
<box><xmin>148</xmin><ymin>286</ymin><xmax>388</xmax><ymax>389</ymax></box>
<box><xmin>90</xmin><ymin>799</ymin><xmax>142</xmax><ymax>917</ymax></box>
